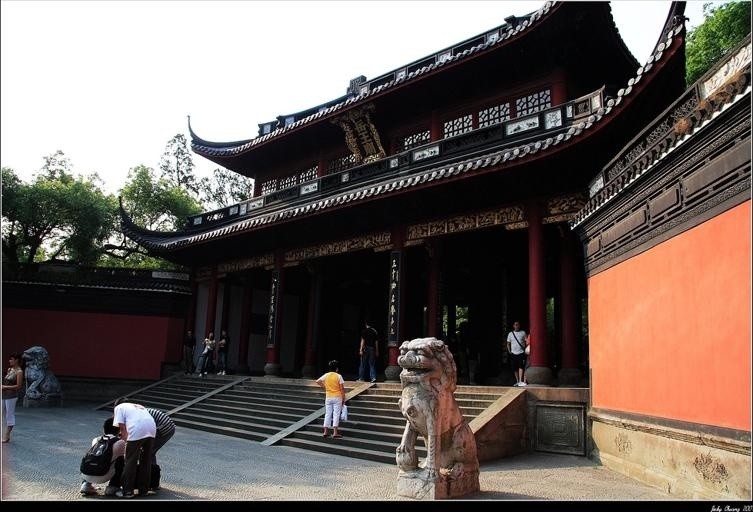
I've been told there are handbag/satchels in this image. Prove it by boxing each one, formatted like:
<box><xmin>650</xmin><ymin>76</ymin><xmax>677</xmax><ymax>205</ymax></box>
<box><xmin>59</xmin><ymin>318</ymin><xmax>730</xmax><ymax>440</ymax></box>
<box><xmin>134</xmin><ymin>464</ymin><xmax>160</xmax><ymax>489</ymax></box>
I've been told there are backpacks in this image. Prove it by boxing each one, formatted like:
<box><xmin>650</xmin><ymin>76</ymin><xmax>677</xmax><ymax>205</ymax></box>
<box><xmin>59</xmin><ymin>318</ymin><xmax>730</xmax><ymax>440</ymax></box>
<box><xmin>80</xmin><ymin>435</ymin><xmax>119</xmax><ymax>476</ymax></box>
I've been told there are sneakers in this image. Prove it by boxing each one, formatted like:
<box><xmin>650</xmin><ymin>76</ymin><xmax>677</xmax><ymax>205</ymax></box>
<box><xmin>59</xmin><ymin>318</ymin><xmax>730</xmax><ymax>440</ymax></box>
<box><xmin>105</xmin><ymin>486</ymin><xmax>134</xmax><ymax>498</ymax></box>
<box><xmin>80</xmin><ymin>482</ymin><xmax>97</xmax><ymax>493</ymax></box>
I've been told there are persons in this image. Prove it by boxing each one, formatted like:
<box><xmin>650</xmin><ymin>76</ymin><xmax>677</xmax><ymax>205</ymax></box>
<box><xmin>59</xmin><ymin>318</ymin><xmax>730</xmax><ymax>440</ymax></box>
<box><xmin>505</xmin><ymin>321</ymin><xmax>528</xmax><ymax>387</ymax></box>
<box><xmin>3</xmin><ymin>351</ymin><xmax>23</xmax><ymax>442</ymax></box>
<box><xmin>523</xmin><ymin>330</ymin><xmax>530</xmax><ymax>382</ymax></box>
<box><xmin>316</xmin><ymin>360</ymin><xmax>346</xmax><ymax>437</ymax></box>
<box><xmin>181</xmin><ymin>329</ymin><xmax>195</xmax><ymax>374</ymax></box>
<box><xmin>217</xmin><ymin>330</ymin><xmax>230</xmax><ymax>376</ymax></box>
<box><xmin>355</xmin><ymin>319</ymin><xmax>379</xmax><ymax>383</ymax></box>
<box><xmin>77</xmin><ymin>396</ymin><xmax>176</xmax><ymax>498</ymax></box>
<box><xmin>198</xmin><ymin>331</ymin><xmax>216</xmax><ymax>377</ymax></box>
